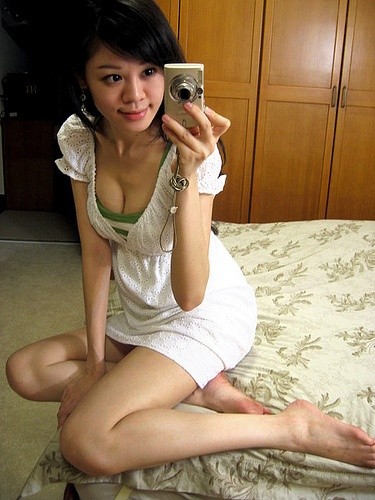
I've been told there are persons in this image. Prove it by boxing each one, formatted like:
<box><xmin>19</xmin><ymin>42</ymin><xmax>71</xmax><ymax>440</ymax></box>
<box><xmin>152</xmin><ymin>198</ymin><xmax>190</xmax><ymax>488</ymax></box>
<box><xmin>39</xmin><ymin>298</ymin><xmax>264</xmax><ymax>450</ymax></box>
<box><xmin>6</xmin><ymin>0</ymin><xmax>374</xmax><ymax>475</ymax></box>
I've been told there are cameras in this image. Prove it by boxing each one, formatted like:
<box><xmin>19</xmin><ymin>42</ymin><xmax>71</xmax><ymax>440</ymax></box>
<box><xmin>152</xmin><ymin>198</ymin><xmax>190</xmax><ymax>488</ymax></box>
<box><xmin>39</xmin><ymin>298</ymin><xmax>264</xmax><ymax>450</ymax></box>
<box><xmin>164</xmin><ymin>63</ymin><xmax>205</xmax><ymax>128</ymax></box>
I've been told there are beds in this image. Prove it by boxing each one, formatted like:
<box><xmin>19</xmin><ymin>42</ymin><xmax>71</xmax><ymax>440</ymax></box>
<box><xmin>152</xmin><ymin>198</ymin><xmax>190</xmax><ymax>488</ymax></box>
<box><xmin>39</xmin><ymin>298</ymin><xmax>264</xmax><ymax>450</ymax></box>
<box><xmin>15</xmin><ymin>219</ymin><xmax>375</xmax><ymax>499</ymax></box>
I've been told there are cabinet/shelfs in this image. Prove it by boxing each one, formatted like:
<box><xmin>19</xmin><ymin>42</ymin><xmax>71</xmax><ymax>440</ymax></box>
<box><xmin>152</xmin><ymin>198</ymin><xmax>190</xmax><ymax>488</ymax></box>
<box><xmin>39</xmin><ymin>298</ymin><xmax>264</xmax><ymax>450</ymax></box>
<box><xmin>249</xmin><ymin>0</ymin><xmax>375</xmax><ymax>224</ymax></box>
<box><xmin>1</xmin><ymin>120</ymin><xmax>55</xmax><ymax>212</ymax></box>
<box><xmin>153</xmin><ymin>0</ymin><xmax>264</xmax><ymax>224</ymax></box>
